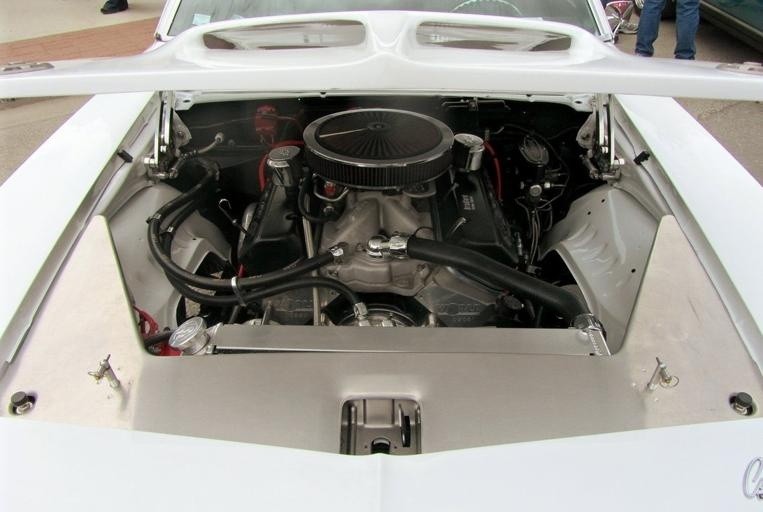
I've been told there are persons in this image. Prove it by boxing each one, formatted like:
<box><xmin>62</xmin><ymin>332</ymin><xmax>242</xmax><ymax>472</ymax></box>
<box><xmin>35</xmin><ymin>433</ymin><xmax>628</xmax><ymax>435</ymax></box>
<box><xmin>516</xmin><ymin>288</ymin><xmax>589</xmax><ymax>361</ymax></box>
<box><xmin>635</xmin><ymin>0</ymin><xmax>700</xmax><ymax>59</ymax></box>
